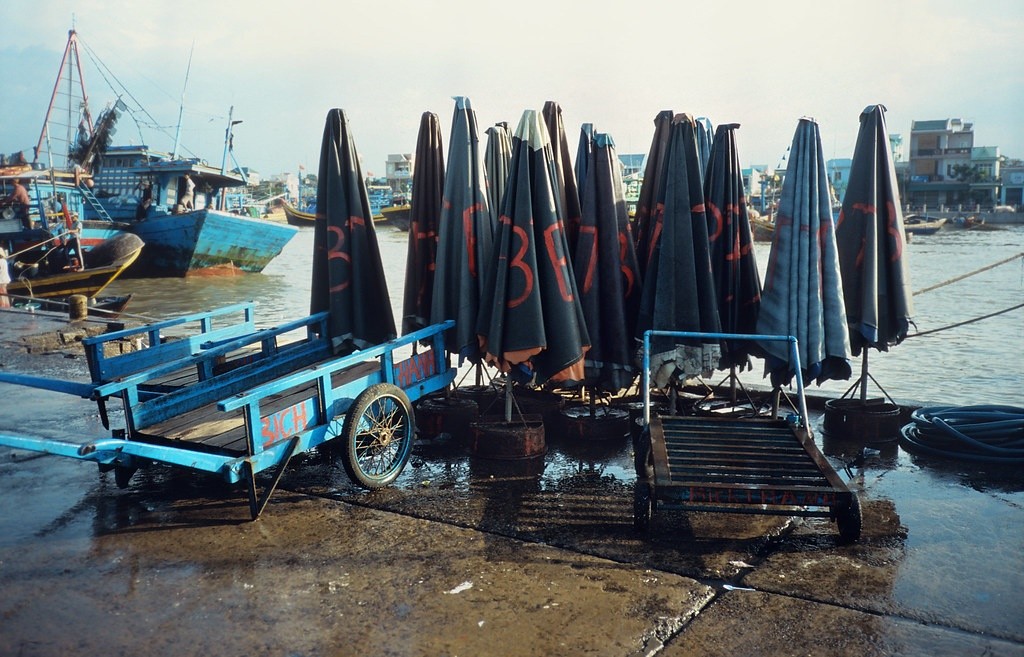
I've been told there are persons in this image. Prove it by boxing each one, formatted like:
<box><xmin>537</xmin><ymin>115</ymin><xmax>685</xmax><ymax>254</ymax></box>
<box><xmin>2</xmin><ymin>178</ymin><xmax>32</xmax><ymax>230</ymax></box>
<box><xmin>62</xmin><ymin>211</ymin><xmax>84</xmax><ymax>272</ymax></box>
<box><xmin>181</xmin><ymin>171</ymin><xmax>196</xmax><ymax>211</ymax></box>
<box><xmin>133</xmin><ymin>180</ymin><xmax>153</xmax><ymax>222</ymax></box>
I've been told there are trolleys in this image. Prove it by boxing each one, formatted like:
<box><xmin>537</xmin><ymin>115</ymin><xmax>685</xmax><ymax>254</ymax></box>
<box><xmin>0</xmin><ymin>302</ymin><xmax>459</xmax><ymax>521</ymax></box>
<box><xmin>630</xmin><ymin>329</ymin><xmax>864</xmax><ymax>542</ymax></box>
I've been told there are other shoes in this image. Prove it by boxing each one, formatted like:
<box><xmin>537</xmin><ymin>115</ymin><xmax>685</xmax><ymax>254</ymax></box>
<box><xmin>183</xmin><ymin>210</ymin><xmax>187</xmax><ymax>211</ymax></box>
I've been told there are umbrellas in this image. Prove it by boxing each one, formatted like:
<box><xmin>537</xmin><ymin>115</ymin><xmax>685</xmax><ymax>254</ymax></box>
<box><xmin>401</xmin><ymin>95</ymin><xmax>915</xmax><ymax>423</ymax></box>
<box><xmin>309</xmin><ymin>108</ymin><xmax>398</xmax><ymax>342</ymax></box>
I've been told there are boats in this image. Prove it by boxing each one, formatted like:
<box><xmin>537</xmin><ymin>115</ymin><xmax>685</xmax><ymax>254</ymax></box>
<box><xmin>749</xmin><ymin>203</ymin><xmax>778</xmax><ymax>244</ymax></box>
<box><xmin>901</xmin><ymin>211</ymin><xmax>949</xmax><ymax>234</ymax></box>
<box><xmin>0</xmin><ymin>43</ymin><xmax>417</xmax><ymax>312</ymax></box>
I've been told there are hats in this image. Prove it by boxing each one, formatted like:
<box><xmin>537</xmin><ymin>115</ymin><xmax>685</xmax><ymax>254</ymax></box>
<box><xmin>142</xmin><ymin>180</ymin><xmax>150</xmax><ymax>185</ymax></box>
<box><xmin>70</xmin><ymin>211</ymin><xmax>79</xmax><ymax>217</ymax></box>
